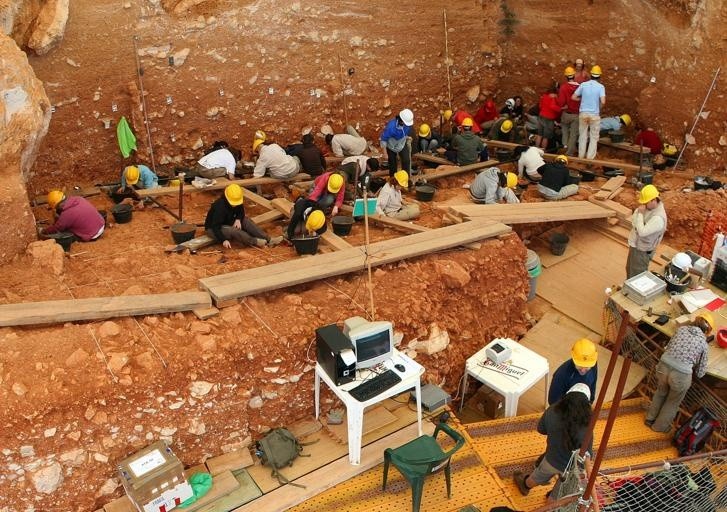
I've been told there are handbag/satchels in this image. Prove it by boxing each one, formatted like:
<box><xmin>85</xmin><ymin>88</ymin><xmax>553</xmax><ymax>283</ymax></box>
<box><xmin>256</xmin><ymin>427</ymin><xmax>299</xmax><ymax>468</ymax></box>
<box><xmin>672</xmin><ymin>405</ymin><xmax>721</xmax><ymax>457</ymax></box>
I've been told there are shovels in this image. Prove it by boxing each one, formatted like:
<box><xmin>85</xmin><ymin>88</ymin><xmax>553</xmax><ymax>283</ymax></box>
<box><xmin>163</xmin><ymin>245</ymin><xmax>224</xmax><ymax>255</ymax></box>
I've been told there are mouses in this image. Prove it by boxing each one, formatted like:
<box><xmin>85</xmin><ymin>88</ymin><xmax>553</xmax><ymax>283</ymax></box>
<box><xmin>394</xmin><ymin>363</ymin><xmax>405</xmax><ymax>372</ymax></box>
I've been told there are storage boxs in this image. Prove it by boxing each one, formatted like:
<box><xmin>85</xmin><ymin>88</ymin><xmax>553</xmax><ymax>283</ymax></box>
<box><xmin>622</xmin><ymin>270</ymin><xmax>667</xmax><ymax>305</ymax></box>
<box><xmin>115</xmin><ymin>437</ymin><xmax>194</xmax><ymax>512</ymax></box>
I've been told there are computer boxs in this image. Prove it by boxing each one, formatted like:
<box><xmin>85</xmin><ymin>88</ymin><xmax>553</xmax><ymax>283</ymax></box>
<box><xmin>315</xmin><ymin>324</ymin><xmax>356</xmax><ymax>386</ymax></box>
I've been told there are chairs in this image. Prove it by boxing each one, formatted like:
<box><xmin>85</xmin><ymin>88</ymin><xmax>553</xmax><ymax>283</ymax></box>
<box><xmin>379</xmin><ymin>420</ymin><xmax>466</xmax><ymax>512</ymax></box>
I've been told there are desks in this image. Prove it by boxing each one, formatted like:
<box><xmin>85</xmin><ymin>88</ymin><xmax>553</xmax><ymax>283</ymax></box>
<box><xmin>312</xmin><ymin>343</ymin><xmax>426</xmax><ymax>465</ymax></box>
<box><xmin>456</xmin><ymin>336</ymin><xmax>549</xmax><ymax>419</ymax></box>
<box><xmin>606</xmin><ymin>258</ymin><xmax>725</xmax><ymax>381</ymax></box>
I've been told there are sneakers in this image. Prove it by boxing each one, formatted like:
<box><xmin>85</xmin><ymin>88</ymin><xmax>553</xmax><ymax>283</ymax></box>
<box><xmin>267</xmin><ymin>236</ymin><xmax>284</xmax><ymax>248</ymax></box>
<box><xmin>257</xmin><ymin>238</ymin><xmax>267</xmax><ymax>248</ymax></box>
<box><xmin>514</xmin><ymin>470</ymin><xmax>529</xmax><ymax>496</ymax></box>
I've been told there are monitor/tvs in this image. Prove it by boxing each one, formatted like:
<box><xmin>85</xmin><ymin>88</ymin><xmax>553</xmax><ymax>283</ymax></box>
<box><xmin>342</xmin><ymin>316</ymin><xmax>393</xmax><ymax>370</ymax></box>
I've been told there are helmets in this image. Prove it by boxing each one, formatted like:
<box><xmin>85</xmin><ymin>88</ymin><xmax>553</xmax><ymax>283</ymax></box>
<box><xmin>566</xmin><ymin>382</ymin><xmax>591</xmax><ymax>402</ymax></box>
<box><xmin>399</xmin><ymin>109</ymin><xmax>414</xmax><ymax>126</ymax></box>
<box><xmin>717</xmin><ymin>327</ymin><xmax>727</xmax><ymax>349</ymax></box>
<box><xmin>47</xmin><ymin>190</ymin><xmax>65</xmax><ymax>209</ymax></box>
<box><xmin>224</xmin><ymin>183</ymin><xmax>244</xmax><ymax>206</ymax></box>
<box><xmin>252</xmin><ymin>138</ymin><xmax>265</xmax><ymax>155</ymax></box>
<box><xmin>564</xmin><ymin>67</ymin><xmax>576</xmax><ymax>76</ymax></box>
<box><xmin>696</xmin><ymin>313</ymin><xmax>715</xmax><ymax>337</ymax></box>
<box><xmin>571</xmin><ymin>338</ymin><xmax>598</xmax><ymax>368</ymax></box>
<box><xmin>638</xmin><ymin>185</ymin><xmax>660</xmax><ymax>204</ymax></box>
<box><xmin>305</xmin><ymin>210</ymin><xmax>325</xmax><ymax>232</ymax></box>
<box><xmin>443</xmin><ymin>110</ymin><xmax>452</xmax><ymax>121</ymax></box>
<box><xmin>662</xmin><ymin>143</ymin><xmax>677</xmax><ymax>156</ymax></box>
<box><xmin>418</xmin><ymin>124</ymin><xmax>430</xmax><ymax>137</ymax></box>
<box><xmin>555</xmin><ymin>154</ymin><xmax>568</xmax><ymax>167</ymax></box>
<box><xmin>394</xmin><ymin>170</ymin><xmax>409</xmax><ymax>188</ymax></box>
<box><xmin>590</xmin><ymin>65</ymin><xmax>603</xmax><ymax>75</ymax></box>
<box><xmin>327</xmin><ymin>174</ymin><xmax>344</xmax><ymax>194</ymax></box>
<box><xmin>125</xmin><ymin>166</ymin><xmax>139</xmax><ymax>185</ymax></box>
<box><xmin>672</xmin><ymin>252</ymin><xmax>692</xmax><ymax>270</ymax></box>
<box><xmin>461</xmin><ymin>118</ymin><xmax>473</xmax><ymax>127</ymax></box>
<box><xmin>500</xmin><ymin>120</ymin><xmax>513</xmax><ymax>134</ymax></box>
<box><xmin>620</xmin><ymin>114</ymin><xmax>632</xmax><ymax>128</ymax></box>
<box><xmin>507</xmin><ymin>172</ymin><xmax>518</xmax><ymax>188</ymax></box>
<box><xmin>485</xmin><ymin>100</ymin><xmax>495</xmax><ymax>113</ymax></box>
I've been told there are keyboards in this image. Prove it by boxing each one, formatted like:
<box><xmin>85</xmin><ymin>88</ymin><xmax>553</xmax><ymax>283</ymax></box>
<box><xmin>348</xmin><ymin>369</ymin><xmax>402</xmax><ymax>402</ymax></box>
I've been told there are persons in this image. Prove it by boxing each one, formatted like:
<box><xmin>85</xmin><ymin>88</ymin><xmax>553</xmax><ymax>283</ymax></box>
<box><xmin>512</xmin><ymin>382</ymin><xmax>593</xmax><ymax>499</ymax></box>
<box><xmin>625</xmin><ymin>184</ymin><xmax>668</xmax><ymax>280</ymax></box>
<box><xmin>644</xmin><ymin>314</ymin><xmax>713</xmax><ymax>433</ymax></box>
<box><xmin>37</xmin><ymin>190</ymin><xmax>106</xmax><ymax>243</ymax></box>
<box><xmin>548</xmin><ymin>338</ymin><xmax>598</xmax><ymax>407</ymax></box>
<box><xmin>194</xmin><ymin>58</ymin><xmax>631</xmax><ymax>251</ymax></box>
<box><xmin>116</xmin><ymin>164</ymin><xmax>162</xmax><ymax>209</ymax></box>
<box><xmin>631</xmin><ymin>122</ymin><xmax>665</xmax><ymax>163</ymax></box>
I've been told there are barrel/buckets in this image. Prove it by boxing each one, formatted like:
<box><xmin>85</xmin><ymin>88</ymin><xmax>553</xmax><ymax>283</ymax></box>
<box><xmin>666</xmin><ymin>266</ymin><xmax>693</xmax><ymax>292</ymax></box>
<box><xmin>156</xmin><ymin>176</ymin><xmax>168</xmax><ymax>184</ymax></box>
<box><xmin>636</xmin><ymin>171</ymin><xmax>653</xmax><ymax>187</ymax></box>
<box><xmin>668</xmin><ymin>151</ymin><xmax>681</xmax><ymax>164</ymax></box>
<box><xmin>608</xmin><ymin>129</ymin><xmax>626</xmax><ymax>143</ymax></box>
<box><xmin>415</xmin><ymin>185</ymin><xmax>435</xmax><ymax>200</ymax></box>
<box><xmin>332</xmin><ymin>215</ymin><xmax>355</xmax><ymax>234</ymax></box>
<box><xmin>242</xmin><ymin>164</ymin><xmax>254</xmax><ymax>173</ymax></box>
<box><xmin>292</xmin><ymin>234</ymin><xmax>319</xmax><ymax>256</ymax></box>
<box><xmin>382</xmin><ymin>160</ymin><xmax>388</xmax><ymax>170</ymax></box>
<box><xmin>109</xmin><ymin>185</ymin><xmax>130</xmax><ymax>203</ymax></box>
<box><xmin>695</xmin><ymin>174</ymin><xmax>713</xmax><ymax>193</ymax></box>
<box><xmin>652</xmin><ymin>159</ymin><xmax>665</xmax><ymax>170</ymax></box>
<box><xmin>604</xmin><ymin>166</ymin><xmax>624</xmax><ymax>178</ymax></box>
<box><xmin>551</xmin><ymin>233</ymin><xmax>569</xmax><ymax>255</ymax></box>
<box><xmin>114</xmin><ymin>204</ymin><xmax>131</xmax><ymax>223</ymax></box>
<box><xmin>55</xmin><ymin>208</ymin><xmax>64</xmax><ymax>225</ymax></box>
<box><xmin>580</xmin><ymin>170</ymin><xmax>595</xmax><ymax>180</ymax></box>
<box><xmin>172</xmin><ymin>223</ymin><xmax>197</xmax><ymax>242</ymax></box>
<box><xmin>95</xmin><ymin>210</ymin><xmax>107</xmax><ymax>227</ymax></box>
<box><xmin>495</xmin><ymin>146</ymin><xmax>510</xmax><ymax>159</ymax></box>
